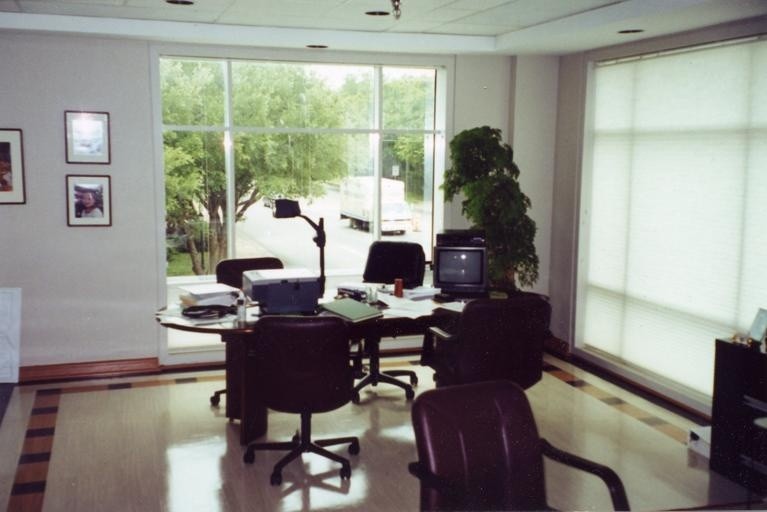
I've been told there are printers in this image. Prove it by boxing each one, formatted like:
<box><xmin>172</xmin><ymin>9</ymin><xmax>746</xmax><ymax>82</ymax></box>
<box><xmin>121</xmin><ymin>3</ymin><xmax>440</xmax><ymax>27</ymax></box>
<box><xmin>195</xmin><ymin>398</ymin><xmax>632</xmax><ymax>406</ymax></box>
<box><xmin>242</xmin><ymin>268</ymin><xmax>321</xmax><ymax>315</ymax></box>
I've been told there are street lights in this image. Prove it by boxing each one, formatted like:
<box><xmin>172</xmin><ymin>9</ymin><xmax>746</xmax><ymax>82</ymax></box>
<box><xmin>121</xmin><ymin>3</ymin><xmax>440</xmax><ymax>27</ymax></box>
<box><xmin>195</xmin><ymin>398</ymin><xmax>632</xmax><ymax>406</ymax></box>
<box><xmin>197</xmin><ymin>213</ymin><xmax>205</xmax><ymax>269</ymax></box>
<box><xmin>298</xmin><ymin>91</ymin><xmax>311</xmax><ymax>197</ymax></box>
<box><xmin>279</xmin><ymin>119</ymin><xmax>292</xmax><ymax>150</ymax></box>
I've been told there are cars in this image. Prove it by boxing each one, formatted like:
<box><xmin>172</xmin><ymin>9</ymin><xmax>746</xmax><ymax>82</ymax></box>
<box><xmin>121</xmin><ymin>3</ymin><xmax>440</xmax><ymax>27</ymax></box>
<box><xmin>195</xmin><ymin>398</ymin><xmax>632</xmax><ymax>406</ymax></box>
<box><xmin>263</xmin><ymin>193</ymin><xmax>286</xmax><ymax>208</ymax></box>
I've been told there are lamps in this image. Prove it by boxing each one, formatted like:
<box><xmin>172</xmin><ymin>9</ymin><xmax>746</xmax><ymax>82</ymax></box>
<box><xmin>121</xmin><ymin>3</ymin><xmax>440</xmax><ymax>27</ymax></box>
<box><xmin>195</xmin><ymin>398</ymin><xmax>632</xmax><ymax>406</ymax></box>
<box><xmin>271</xmin><ymin>198</ymin><xmax>330</xmax><ymax>296</ymax></box>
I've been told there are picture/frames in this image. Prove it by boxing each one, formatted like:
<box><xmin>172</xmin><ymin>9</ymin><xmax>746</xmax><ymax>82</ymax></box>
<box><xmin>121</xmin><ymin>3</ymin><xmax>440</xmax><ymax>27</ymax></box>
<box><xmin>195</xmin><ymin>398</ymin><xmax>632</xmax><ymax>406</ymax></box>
<box><xmin>0</xmin><ymin>128</ymin><xmax>29</xmax><ymax>206</ymax></box>
<box><xmin>65</xmin><ymin>172</ymin><xmax>112</xmax><ymax>228</ymax></box>
<box><xmin>63</xmin><ymin>109</ymin><xmax>112</xmax><ymax>166</ymax></box>
<box><xmin>0</xmin><ymin>288</ymin><xmax>23</xmax><ymax>384</ymax></box>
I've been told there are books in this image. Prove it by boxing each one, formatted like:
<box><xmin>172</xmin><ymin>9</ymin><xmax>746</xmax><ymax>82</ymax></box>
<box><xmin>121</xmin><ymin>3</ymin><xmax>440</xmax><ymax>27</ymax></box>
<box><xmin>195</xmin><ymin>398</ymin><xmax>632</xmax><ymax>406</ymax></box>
<box><xmin>319</xmin><ymin>297</ymin><xmax>384</xmax><ymax>324</ymax></box>
<box><xmin>176</xmin><ymin>282</ymin><xmax>241</xmax><ymax>320</ymax></box>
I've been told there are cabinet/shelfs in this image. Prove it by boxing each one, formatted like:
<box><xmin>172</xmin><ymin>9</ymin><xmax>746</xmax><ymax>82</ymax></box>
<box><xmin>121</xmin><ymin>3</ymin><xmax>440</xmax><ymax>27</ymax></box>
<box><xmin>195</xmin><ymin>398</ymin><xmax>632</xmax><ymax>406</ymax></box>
<box><xmin>705</xmin><ymin>331</ymin><xmax>767</xmax><ymax>500</ymax></box>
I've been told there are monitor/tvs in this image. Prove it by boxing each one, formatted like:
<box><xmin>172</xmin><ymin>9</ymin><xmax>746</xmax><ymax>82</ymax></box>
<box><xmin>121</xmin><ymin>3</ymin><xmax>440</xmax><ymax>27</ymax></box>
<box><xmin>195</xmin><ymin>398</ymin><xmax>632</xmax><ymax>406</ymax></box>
<box><xmin>434</xmin><ymin>246</ymin><xmax>487</xmax><ymax>302</ymax></box>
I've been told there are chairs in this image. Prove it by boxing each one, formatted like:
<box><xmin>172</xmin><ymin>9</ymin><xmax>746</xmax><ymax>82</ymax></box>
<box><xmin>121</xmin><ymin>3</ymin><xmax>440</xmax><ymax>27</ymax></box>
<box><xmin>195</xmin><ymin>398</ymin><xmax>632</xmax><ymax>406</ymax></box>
<box><xmin>241</xmin><ymin>310</ymin><xmax>361</xmax><ymax>487</ymax></box>
<box><xmin>342</xmin><ymin>240</ymin><xmax>425</xmax><ymax>405</ymax></box>
<box><xmin>414</xmin><ymin>287</ymin><xmax>548</xmax><ymax>390</ymax></box>
<box><xmin>401</xmin><ymin>378</ymin><xmax>634</xmax><ymax>512</ymax></box>
<box><xmin>214</xmin><ymin>255</ymin><xmax>283</xmax><ymax>290</ymax></box>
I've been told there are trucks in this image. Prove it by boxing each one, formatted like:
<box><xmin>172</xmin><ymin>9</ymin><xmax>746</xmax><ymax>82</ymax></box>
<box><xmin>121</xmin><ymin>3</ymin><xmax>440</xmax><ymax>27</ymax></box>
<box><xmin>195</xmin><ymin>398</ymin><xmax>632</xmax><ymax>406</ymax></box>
<box><xmin>338</xmin><ymin>177</ymin><xmax>410</xmax><ymax>236</ymax></box>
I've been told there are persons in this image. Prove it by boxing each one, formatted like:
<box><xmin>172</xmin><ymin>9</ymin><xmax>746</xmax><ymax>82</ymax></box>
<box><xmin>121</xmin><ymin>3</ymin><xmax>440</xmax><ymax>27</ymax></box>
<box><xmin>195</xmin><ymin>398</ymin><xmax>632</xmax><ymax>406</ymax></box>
<box><xmin>0</xmin><ymin>157</ymin><xmax>13</xmax><ymax>191</ymax></box>
<box><xmin>79</xmin><ymin>189</ymin><xmax>102</xmax><ymax>218</ymax></box>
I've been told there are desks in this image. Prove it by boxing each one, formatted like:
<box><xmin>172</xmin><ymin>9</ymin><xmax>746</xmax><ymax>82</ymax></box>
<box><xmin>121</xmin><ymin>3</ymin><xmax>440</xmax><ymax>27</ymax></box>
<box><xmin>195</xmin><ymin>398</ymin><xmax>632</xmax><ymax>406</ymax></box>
<box><xmin>156</xmin><ymin>281</ymin><xmax>549</xmax><ymax>442</ymax></box>
